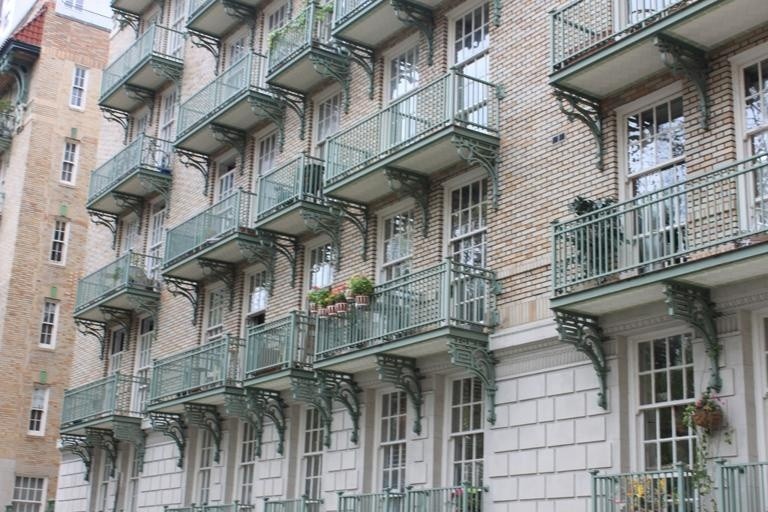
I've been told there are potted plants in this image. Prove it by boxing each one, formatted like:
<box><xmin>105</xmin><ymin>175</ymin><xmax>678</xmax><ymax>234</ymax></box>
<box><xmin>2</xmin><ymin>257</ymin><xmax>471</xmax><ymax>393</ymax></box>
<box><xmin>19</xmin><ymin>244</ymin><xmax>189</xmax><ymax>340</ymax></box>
<box><xmin>309</xmin><ymin>286</ymin><xmax>353</xmax><ymax>317</ymax></box>
<box><xmin>351</xmin><ymin>273</ymin><xmax>371</xmax><ymax>309</ymax></box>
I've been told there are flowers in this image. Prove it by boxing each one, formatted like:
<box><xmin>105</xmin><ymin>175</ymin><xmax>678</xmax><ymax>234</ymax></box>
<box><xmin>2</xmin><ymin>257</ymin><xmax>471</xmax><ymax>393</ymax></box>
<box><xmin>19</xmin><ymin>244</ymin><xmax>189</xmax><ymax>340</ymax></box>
<box><xmin>682</xmin><ymin>383</ymin><xmax>724</xmax><ymax>428</ymax></box>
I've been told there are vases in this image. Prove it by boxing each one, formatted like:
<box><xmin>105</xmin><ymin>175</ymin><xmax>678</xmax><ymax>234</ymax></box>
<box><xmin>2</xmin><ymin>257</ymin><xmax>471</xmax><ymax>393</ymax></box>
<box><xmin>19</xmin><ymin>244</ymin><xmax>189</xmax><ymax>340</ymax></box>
<box><xmin>690</xmin><ymin>410</ymin><xmax>723</xmax><ymax>427</ymax></box>
